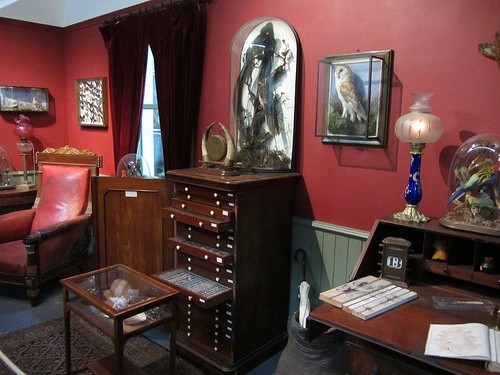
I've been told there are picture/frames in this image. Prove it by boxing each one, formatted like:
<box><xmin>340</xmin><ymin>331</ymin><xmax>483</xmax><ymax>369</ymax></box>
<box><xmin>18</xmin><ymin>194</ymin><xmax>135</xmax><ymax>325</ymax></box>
<box><xmin>314</xmin><ymin>49</ymin><xmax>394</xmax><ymax>148</ymax></box>
<box><xmin>75</xmin><ymin>76</ymin><xmax>108</xmax><ymax>127</ymax></box>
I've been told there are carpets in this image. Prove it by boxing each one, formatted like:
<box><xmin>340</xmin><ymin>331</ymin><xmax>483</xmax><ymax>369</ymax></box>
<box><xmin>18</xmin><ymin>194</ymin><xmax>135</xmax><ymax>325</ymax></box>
<box><xmin>0</xmin><ymin>312</ymin><xmax>205</xmax><ymax>375</ymax></box>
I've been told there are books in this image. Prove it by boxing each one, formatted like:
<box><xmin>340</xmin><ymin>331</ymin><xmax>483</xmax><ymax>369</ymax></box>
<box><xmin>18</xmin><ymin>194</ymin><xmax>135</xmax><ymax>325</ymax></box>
<box><xmin>424</xmin><ymin>323</ymin><xmax>500</xmax><ymax>373</ymax></box>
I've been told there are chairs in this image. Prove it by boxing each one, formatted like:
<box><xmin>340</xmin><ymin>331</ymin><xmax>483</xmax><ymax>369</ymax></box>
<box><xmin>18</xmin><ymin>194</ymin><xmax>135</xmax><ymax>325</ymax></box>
<box><xmin>0</xmin><ymin>152</ymin><xmax>102</xmax><ymax>308</ymax></box>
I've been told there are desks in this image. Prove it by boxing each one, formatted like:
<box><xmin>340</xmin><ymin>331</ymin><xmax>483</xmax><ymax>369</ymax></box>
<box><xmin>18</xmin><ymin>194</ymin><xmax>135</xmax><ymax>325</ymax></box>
<box><xmin>59</xmin><ymin>263</ymin><xmax>182</xmax><ymax>375</ymax></box>
<box><xmin>0</xmin><ymin>185</ymin><xmax>37</xmax><ymax>206</ymax></box>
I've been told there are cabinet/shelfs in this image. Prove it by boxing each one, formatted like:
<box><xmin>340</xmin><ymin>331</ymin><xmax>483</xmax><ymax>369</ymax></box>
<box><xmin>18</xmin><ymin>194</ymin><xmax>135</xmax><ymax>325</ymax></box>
<box><xmin>305</xmin><ymin>209</ymin><xmax>500</xmax><ymax>375</ymax></box>
<box><xmin>152</xmin><ymin>168</ymin><xmax>303</xmax><ymax>375</ymax></box>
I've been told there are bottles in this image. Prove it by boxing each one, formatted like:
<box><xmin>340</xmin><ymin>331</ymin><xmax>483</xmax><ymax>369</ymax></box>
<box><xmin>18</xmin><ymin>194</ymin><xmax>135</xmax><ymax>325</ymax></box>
<box><xmin>480</xmin><ymin>256</ymin><xmax>496</xmax><ymax>273</ymax></box>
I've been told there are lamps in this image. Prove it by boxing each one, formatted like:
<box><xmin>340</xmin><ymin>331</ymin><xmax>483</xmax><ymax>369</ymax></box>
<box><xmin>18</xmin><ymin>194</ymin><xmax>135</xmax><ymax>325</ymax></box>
<box><xmin>14</xmin><ymin>113</ymin><xmax>36</xmax><ymax>191</ymax></box>
<box><xmin>393</xmin><ymin>92</ymin><xmax>443</xmax><ymax>224</ymax></box>
